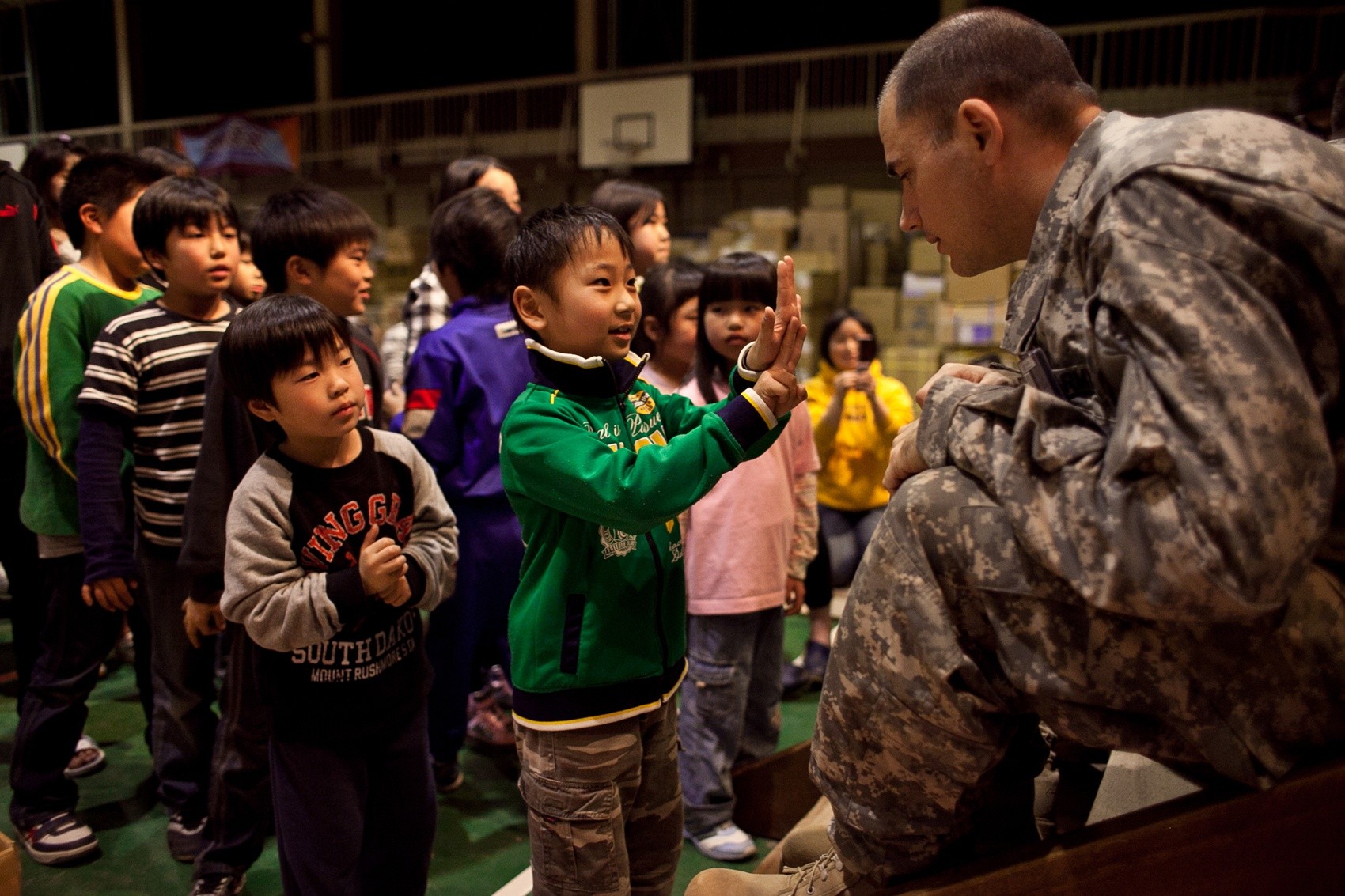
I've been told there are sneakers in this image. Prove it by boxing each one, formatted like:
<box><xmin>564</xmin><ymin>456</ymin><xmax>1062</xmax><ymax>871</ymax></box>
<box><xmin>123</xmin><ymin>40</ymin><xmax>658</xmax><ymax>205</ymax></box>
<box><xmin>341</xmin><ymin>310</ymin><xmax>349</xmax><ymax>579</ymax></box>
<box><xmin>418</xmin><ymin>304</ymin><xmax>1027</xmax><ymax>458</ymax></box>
<box><xmin>684</xmin><ymin>818</ymin><xmax>758</xmax><ymax>863</ymax></box>
<box><xmin>165</xmin><ymin>808</ymin><xmax>212</xmax><ymax>864</ymax></box>
<box><xmin>439</xmin><ymin>759</ymin><xmax>465</xmax><ymax>793</ymax></box>
<box><xmin>186</xmin><ymin>869</ymin><xmax>247</xmax><ymax>896</ymax></box>
<box><xmin>16</xmin><ymin>810</ymin><xmax>102</xmax><ymax>867</ymax></box>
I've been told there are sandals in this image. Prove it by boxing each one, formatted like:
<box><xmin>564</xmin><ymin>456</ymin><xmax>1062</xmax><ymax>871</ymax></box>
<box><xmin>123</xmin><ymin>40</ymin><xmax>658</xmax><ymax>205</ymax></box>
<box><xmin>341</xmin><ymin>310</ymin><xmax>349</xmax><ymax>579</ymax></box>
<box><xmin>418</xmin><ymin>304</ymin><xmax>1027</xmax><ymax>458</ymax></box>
<box><xmin>64</xmin><ymin>733</ymin><xmax>107</xmax><ymax>780</ymax></box>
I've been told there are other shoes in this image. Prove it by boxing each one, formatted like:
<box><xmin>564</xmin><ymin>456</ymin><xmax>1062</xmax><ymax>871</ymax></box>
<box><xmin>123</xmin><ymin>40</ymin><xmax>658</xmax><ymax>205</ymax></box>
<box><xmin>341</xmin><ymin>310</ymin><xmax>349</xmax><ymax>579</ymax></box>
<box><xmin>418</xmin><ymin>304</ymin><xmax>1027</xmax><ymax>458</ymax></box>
<box><xmin>682</xmin><ymin>844</ymin><xmax>852</xmax><ymax>896</ymax></box>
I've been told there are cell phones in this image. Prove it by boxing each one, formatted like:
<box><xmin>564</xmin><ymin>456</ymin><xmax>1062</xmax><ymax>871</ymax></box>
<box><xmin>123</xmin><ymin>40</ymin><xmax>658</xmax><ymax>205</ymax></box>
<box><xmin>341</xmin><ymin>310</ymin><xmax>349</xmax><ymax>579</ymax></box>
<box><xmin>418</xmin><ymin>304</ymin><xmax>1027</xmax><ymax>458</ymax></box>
<box><xmin>854</xmin><ymin>340</ymin><xmax>877</xmax><ymax>373</ymax></box>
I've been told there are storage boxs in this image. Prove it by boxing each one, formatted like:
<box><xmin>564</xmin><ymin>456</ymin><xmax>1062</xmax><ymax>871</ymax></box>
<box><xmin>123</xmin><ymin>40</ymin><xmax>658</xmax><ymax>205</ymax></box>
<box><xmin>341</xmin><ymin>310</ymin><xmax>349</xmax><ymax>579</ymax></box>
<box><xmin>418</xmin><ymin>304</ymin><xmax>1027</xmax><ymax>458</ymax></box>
<box><xmin>366</xmin><ymin>182</ymin><xmax>1028</xmax><ymax>408</ymax></box>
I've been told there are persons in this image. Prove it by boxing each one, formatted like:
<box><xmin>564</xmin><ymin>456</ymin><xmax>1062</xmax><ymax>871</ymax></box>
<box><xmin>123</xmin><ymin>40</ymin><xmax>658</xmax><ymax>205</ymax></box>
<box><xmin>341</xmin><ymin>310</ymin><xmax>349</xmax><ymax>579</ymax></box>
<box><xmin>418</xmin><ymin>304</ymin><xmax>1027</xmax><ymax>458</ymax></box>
<box><xmin>793</xmin><ymin>312</ymin><xmax>924</xmax><ymax>681</ymax></box>
<box><xmin>590</xmin><ymin>184</ymin><xmax>680</xmax><ymax>272</ymax></box>
<box><xmin>627</xmin><ymin>262</ymin><xmax>713</xmax><ymax>412</ymax></box>
<box><xmin>395</xmin><ymin>194</ymin><xmax>532</xmax><ymax>794</ymax></box>
<box><xmin>684</xmin><ymin>0</ymin><xmax>1344</xmax><ymax>895</ymax></box>
<box><xmin>0</xmin><ymin>141</ymin><xmax>526</xmax><ymax>896</ymax></box>
<box><xmin>501</xmin><ymin>207</ymin><xmax>809</xmax><ymax>896</ymax></box>
